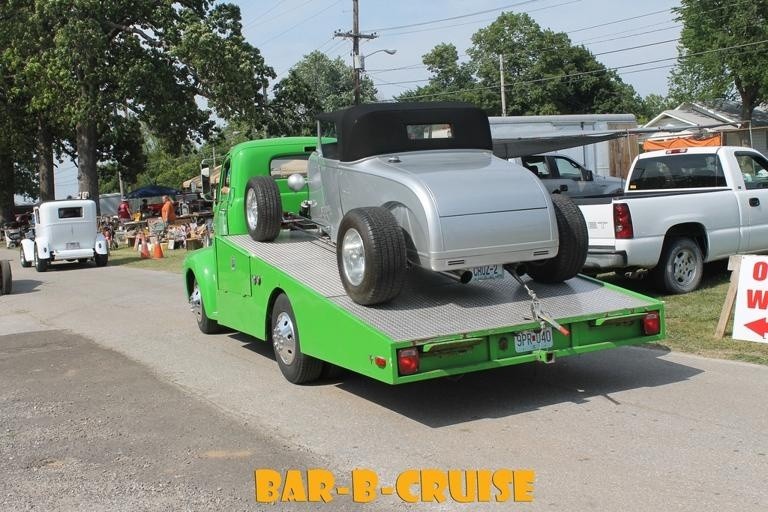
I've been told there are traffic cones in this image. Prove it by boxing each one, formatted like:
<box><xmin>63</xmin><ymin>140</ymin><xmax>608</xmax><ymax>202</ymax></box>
<box><xmin>151</xmin><ymin>234</ymin><xmax>165</xmax><ymax>258</ymax></box>
<box><xmin>140</xmin><ymin>231</ymin><xmax>150</xmax><ymax>260</ymax></box>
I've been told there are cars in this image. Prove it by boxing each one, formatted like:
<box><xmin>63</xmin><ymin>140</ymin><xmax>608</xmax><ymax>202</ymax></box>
<box><xmin>0</xmin><ymin>257</ymin><xmax>13</xmax><ymax>295</ymax></box>
<box><xmin>17</xmin><ymin>197</ymin><xmax>109</xmax><ymax>273</ymax></box>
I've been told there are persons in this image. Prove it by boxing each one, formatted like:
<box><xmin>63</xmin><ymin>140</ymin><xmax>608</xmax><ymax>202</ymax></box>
<box><xmin>63</xmin><ymin>196</ymin><xmax>77</xmax><ymax>214</ymax></box>
<box><xmin>161</xmin><ymin>195</ymin><xmax>177</xmax><ymax>229</ymax></box>
<box><xmin>739</xmin><ymin>138</ymin><xmax>753</xmax><ymax>174</ymax></box>
<box><xmin>102</xmin><ymin>216</ymin><xmax>113</xmax><ymax>252</ymax></box>
<box><xmin>139</xmin><ymin>199</ymin><xmax>148</xmax><ymax>214</ymax></box>
<box><xmin>117</xmin><ymin>196</ymin><xmax>133</xmax><ymax>224</ymax></box>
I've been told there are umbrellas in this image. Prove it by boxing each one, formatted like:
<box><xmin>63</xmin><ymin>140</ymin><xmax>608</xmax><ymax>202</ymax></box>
<box><xmin>122</xmin><ymin>185</ymin><xmax>183</xmax><ymax>201</ymax></box>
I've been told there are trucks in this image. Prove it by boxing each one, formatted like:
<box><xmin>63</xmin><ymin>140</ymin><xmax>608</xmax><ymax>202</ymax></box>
<box><xmin>177</xmin><ymin>97</ymin><xmax>767</xmax><ymax>396</ymax></box>
